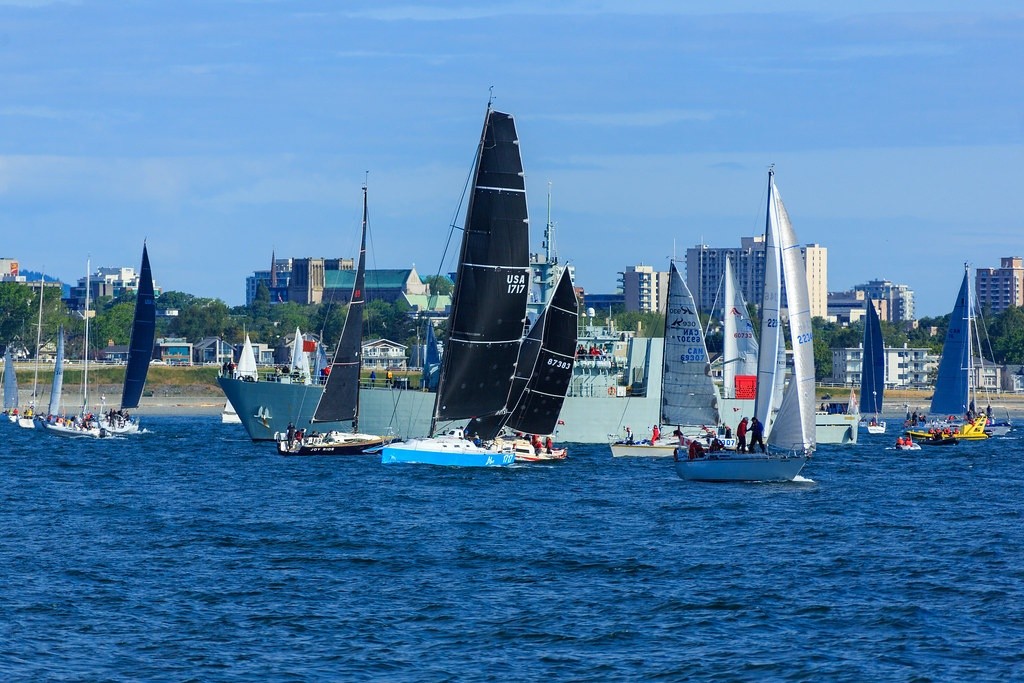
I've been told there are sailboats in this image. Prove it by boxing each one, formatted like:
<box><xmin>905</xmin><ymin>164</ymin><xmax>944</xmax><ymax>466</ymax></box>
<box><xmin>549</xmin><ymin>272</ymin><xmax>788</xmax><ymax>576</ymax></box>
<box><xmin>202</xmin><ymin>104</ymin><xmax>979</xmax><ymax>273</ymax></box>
<box><xmin>214</xmin><ymin>86</ymin><xmax>1013</xmax><ymax>484</ymax></box>
<box><xmin>0</xmin><ymin>238</ymin><xmax>157</xmax><ymax>438</ymax></box>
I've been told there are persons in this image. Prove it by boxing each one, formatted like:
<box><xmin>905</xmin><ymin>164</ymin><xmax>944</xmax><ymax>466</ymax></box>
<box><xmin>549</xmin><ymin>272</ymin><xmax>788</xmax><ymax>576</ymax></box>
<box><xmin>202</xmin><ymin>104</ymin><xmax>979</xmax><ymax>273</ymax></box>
<box><xmin>320</xmin><ymin>366</ymin><xmax>330</xmax><ymax>384</ymax></box>
<box><xmin>821</xmin><ymin>403</ymin><xmax>831</xmax><ymax>413</ymax></box>
<box><xmin>929</xmin><ymin>427</ymin><xmax>958</xmax><ymax>440</ymax></box>
<box><xmin>513</xmin><ymin>432</ymin><xmax>553</xmax><ymax>454</ymax></box>
<box><xmin>872</xmin><ymin>416</ymin><xmax>876</xmax><ymax>423</ymax></box>
<box><xmin>966</xmin><ymin>410</ymin><xmax>973</xmax><ymax>421</ymax></box>
<box><xmin>736</xmin><ymin>418</ymin><xmax>750</xmax><ymax>452</ymax></box>
<box><xmin>370</xmin><ymin>371</ymin><xmax>375</xmax><ymax>387</ymax></box>
<box><xmin>747</xmin><ymin>417</ymin><xmax>766</xmax><ymax>453</ymax></box>
<box><xmin>625</xmin><ymin>427</ymin><xmax>633</xmax><ymax>445</ymax></box>
<box><xmin>474</xmin><ymin>435</ymin><xmax>481</xmax><ymax>446</ymax></box>
<box><xmin>275</xmin><ymin>365</ymin><xmax>290</xmax><ymax>377</ymax></box>
<box><xmin>286</xmin><ymin>422</ymin><xmax>333</xmax><ymax>449</ymax></box>
<box><xmin>222</xmin><ymin>362</ymin><xmax>238</xmax><ymax>378</ymax></box>
<box><xmin>386</xmin><ymin>369</ymin><xmax>393</xmax><ymax>387</ymax></box>
<box><xmin>907</xmin><ymin>411</ymin><xmax>926</xmax><ymax>426</ymax></box>
<box><xmin>4</xmin><ymin>406</ymin><xmax>130</xmax><ymax>431</ymax></box>
<box><xmin>575</xmin><ymin>343</ymin><xmax>607</xmax><ymax>361</ymax></box>
<box><xmin>897</xmin><ymin>436</ymin><xmax>913</xmax><ymax>447</ymax></box>
<box><xmin>690</xmin><ymin>423</ymin><xmax>732</xmax><ymax>459</ymax></box>
<box><xmin>651</xmin><ymin>425</ymin><xmax>659</xmax><ymax>445</ymax></box>
<box><xmin>987</xmin><ymin>405</ymin><xmax>993</xmax><ymax>418</ymax></box>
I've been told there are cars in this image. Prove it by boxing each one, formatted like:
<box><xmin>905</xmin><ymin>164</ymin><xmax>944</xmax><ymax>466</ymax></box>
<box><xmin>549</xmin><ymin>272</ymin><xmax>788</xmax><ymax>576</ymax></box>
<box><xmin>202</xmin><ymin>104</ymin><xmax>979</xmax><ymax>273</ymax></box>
<box><xmin>149</xmin><ymin>358</ymin><xmax>166</xmax><ymax>366</ymax></box>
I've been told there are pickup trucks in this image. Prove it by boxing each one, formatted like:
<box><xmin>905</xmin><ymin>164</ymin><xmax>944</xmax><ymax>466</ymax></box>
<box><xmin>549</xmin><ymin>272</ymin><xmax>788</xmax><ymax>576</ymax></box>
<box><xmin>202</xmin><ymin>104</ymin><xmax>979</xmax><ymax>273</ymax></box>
<box><xmin>44</xmin><ymin>355</ymin><xmax>70</xmax><ymax>364</ymax></box>
